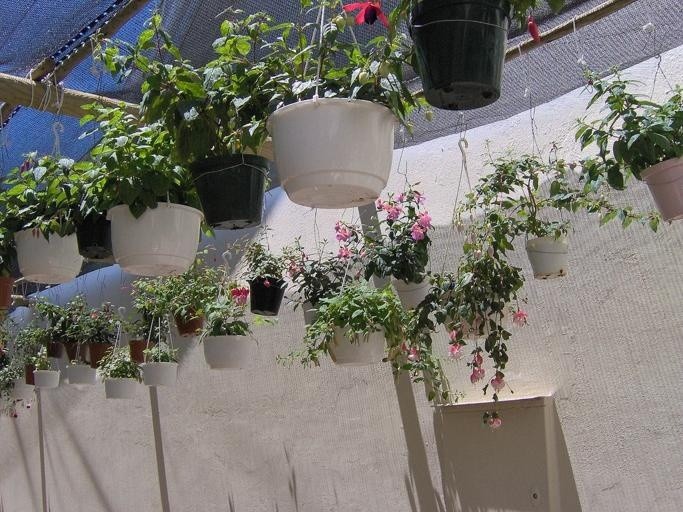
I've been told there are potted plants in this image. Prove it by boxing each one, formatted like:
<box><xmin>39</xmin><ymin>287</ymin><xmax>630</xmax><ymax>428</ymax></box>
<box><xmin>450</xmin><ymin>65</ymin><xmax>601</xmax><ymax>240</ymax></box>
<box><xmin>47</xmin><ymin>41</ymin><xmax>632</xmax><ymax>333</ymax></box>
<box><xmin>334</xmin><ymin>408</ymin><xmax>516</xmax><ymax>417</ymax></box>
<box><xmin>0</xmin><ymin>0</ymin><xmax>683</xmax><ymax>432</ymax></box>
